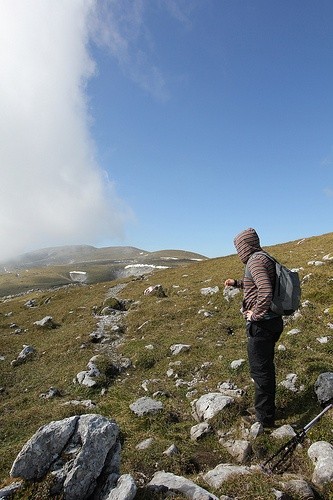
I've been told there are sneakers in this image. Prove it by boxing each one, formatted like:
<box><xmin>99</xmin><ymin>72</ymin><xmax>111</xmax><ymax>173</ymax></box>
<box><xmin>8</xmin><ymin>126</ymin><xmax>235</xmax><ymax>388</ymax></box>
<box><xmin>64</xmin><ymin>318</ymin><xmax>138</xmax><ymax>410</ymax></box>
<box><xmin>247</xmin><ymin>407</ymin><xmax>257</xmax><ymax>415</ymax></box>
<box><xmin>242</xmin><ymin>415</ymin><xmax>274</xmax><ymax>432</ymax></box>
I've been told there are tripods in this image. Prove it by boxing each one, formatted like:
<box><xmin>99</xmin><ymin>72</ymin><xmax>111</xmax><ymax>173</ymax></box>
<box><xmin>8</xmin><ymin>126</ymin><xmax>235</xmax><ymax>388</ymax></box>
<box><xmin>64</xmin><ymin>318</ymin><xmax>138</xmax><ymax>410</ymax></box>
<box><xmin>262</xmin><ymin>404</ymin><xmax>333</xmax><ymax>474</ymax></box>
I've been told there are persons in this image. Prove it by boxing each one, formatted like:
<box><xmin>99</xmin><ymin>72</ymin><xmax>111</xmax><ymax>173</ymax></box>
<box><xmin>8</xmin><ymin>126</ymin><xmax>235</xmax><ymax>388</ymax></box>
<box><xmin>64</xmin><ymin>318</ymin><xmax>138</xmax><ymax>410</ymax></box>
<box><xmin>224</xmin><ymin>227</ymin><xmax>284</xmax><ymax>428</ymax></box>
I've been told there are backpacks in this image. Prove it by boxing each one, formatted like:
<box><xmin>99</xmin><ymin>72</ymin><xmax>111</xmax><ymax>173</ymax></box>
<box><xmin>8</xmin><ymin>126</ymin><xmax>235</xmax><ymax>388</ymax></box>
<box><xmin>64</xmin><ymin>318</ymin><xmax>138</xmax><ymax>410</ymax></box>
<box><xmin>254</xmin><ymin>251</ymin><xmax>302</xmax><ymax>316</ymax></box>
